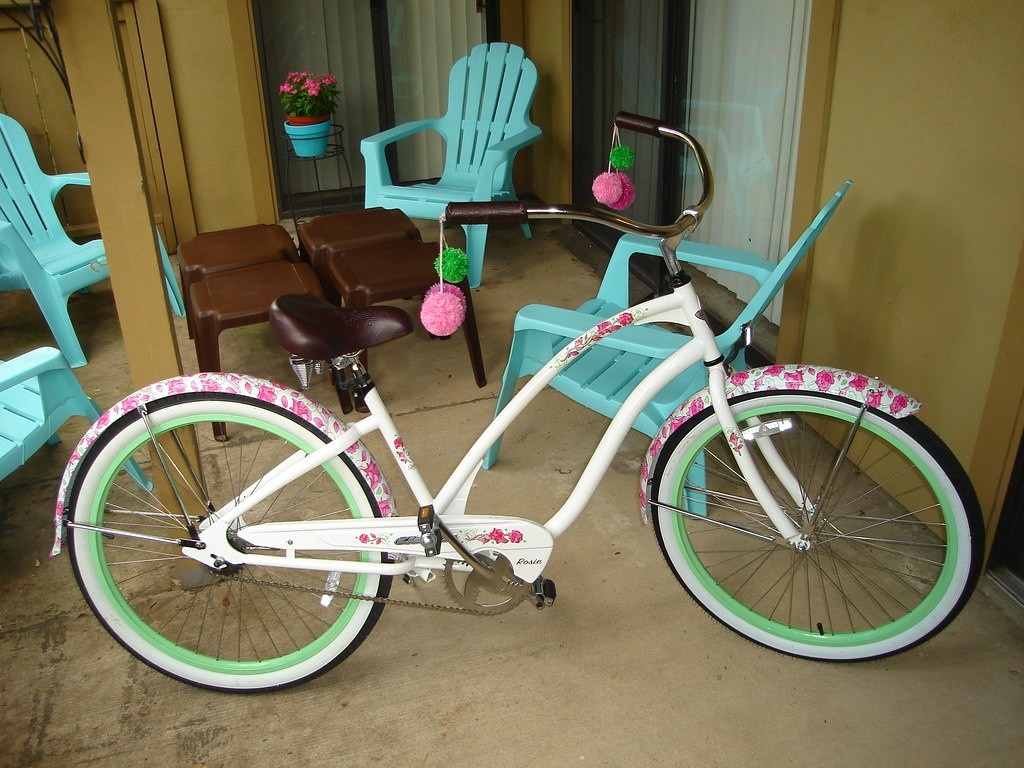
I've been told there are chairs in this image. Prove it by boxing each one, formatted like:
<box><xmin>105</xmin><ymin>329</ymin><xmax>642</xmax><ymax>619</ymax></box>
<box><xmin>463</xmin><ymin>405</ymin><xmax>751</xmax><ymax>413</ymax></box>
<box><xmin>360</xmin><ymin>42</ymin><xmax>543</xmax><ymax>288</ymax></box>
<box><xmin>482</xmin><ymin>180</ymin><xmax>851</xmax><ymax>520</ymax></box>
<box><xmin>0</xmin><ymin>347</ymin><xmax>154</xmax><ymax>495</ymax></box>
<box><xmin>0</xmin><ymin>114</ymin><xmax>186</xmax><ymax>368</ymax></box>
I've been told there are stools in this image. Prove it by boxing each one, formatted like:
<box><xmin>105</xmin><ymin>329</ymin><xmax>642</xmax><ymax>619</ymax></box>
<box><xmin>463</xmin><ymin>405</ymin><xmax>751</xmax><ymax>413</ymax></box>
<box><xmin>296</xmin><ymin>207</ymin><xmax>452</xmax><ymax>340</ymax></box>
<box><xmin>185</xmin><ymin>260</ymin><xmax>353</xmax><ymax>443</ymax></box>
<box><xmin>321</xmin><ymin>242</ymin><xmax>487</xmax><ymax>414</ymax></box>
<box><xmin>177</xmin><ymin>224</ymin><xmax>298</xmax><ymax>340</ymax></box>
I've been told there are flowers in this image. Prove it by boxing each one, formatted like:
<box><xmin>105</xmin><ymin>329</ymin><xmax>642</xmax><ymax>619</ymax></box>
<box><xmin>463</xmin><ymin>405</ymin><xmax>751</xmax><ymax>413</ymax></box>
<box><xmin>278</xmin><ymin>70</ymin><xmax>342</xmax><ymax>116</ymax></box>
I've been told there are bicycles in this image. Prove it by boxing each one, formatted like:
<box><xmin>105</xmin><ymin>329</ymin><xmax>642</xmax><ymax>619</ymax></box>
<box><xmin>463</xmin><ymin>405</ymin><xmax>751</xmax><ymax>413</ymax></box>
<box><xmin>46</xmin><ymin>111</ymin><xmax>990</xmax><ymax>696</ymax></box>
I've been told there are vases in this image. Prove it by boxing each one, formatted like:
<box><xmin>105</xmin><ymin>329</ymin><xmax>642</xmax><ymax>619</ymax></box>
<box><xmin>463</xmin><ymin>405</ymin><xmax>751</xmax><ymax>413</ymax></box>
<box><xmin>284</xmin><ymin>113</ymin><xmax>330</xmax><ymax>127</ymax></box>
<box><xmin>284</xmin><ymin>121</ymin><xmax>331</xmax><ymax>157</ymax></box>
